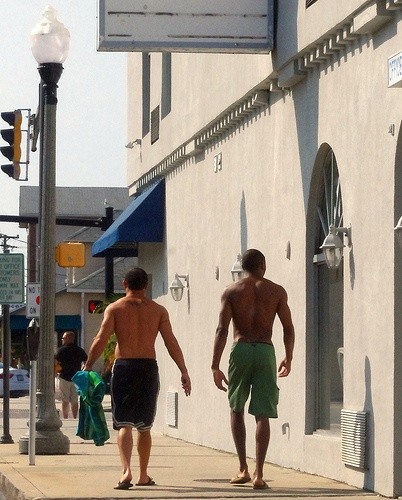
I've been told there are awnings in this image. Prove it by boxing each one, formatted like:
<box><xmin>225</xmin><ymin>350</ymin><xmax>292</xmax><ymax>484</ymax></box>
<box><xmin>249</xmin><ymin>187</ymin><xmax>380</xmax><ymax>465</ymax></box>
<box><xmin>91</xmin><ymin>178</ymin><xmax>166</xmax><ymax>257</ymax></box>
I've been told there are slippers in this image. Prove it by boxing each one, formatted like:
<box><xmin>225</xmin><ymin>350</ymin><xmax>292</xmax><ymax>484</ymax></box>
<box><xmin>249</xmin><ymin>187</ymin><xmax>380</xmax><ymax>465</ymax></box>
<box><xmin>113</xmin><ymin>480</ymin><xmax>133</xmax><ymax>489</ymax></box>
<box><xmin>135</xmin><ymin>476</ymin><xmax>155</xmax><ymax>486</ymax></box>
<box><xmin>230</xmin><ymin>475</ymin><xmax>251</xmax><ymax>484</ymax></box>
<box><xmin>253</xmin><ymin>480</ymin><xmax>271</xmax><ymax>489</ymax></box>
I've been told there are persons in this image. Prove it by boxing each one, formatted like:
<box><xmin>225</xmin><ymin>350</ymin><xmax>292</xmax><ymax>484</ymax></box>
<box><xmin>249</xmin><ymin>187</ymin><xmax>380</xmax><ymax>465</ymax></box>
<box><xmin>54</xmin><ymin>331</ymin><xmax>88</xmax><ymax>419</ymax></box>
<box><xmin>83</xmin><ymin>267</ymin><xmax>191</xmax><ymax>489</ymax></box>
<box><xmin>211</xmin><ymin>249</ymin><xmax>295</xmax><ymax>489</ymax></box>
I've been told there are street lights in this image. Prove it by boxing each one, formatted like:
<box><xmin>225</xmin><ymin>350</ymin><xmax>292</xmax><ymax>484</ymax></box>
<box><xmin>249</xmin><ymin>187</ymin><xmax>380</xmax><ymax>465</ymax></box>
<box><xmin>16</xmin><ymin>6</ymin><xmax>70</xmax><ymax>456</ymax></box>
